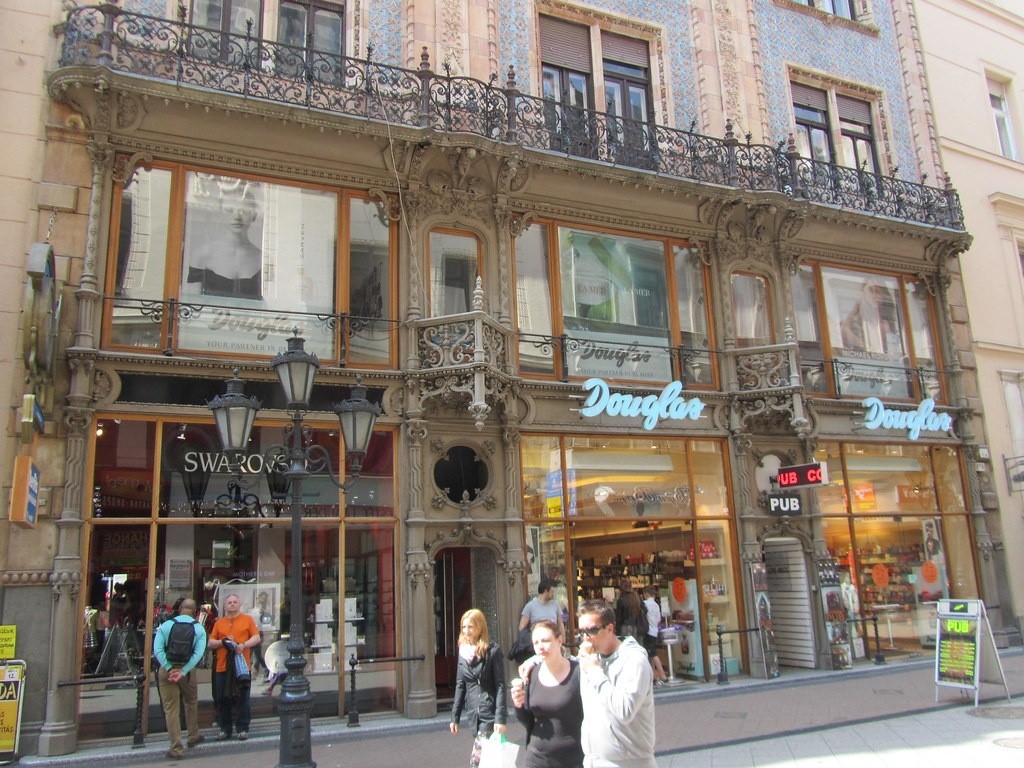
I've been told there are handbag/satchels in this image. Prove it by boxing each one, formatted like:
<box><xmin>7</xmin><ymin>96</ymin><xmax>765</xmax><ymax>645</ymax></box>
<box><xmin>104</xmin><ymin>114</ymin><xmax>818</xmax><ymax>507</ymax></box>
<box><xmin>479</xmin><ymin>731</ymin><xmax>520</xmax><ymax>768</ymax></box>
<box><xmin>637</xmin><ymin>609</ymin><xmax>649</xmax><ymax>634</ymax></box>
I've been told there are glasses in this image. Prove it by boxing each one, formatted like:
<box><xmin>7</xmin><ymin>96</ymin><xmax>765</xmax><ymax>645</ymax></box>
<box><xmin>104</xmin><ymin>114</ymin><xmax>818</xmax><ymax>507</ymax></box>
<box><xmin>576</xmin><ymin>624</ymin><xmax>607</xmax><ymax>636</ymax></box>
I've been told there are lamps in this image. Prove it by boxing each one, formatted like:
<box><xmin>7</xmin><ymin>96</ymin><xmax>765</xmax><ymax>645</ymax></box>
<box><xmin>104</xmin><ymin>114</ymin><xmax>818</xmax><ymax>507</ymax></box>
<box><xmin>630</xmin><ymin>494</ymin><xmax>649</xmax><ymax>528</ymax></box>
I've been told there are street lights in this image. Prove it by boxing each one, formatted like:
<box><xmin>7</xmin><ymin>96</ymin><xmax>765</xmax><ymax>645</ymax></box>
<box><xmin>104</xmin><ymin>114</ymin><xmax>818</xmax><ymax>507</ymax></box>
<box><xmin>205</xmin><ymin>326</ymin><xmax>383</xmax><ymax>768</ymax></box>
<box><xmin>179</xmin><ymin>443</ymin><xmax>292</xmax><ymax>584</ymax></box>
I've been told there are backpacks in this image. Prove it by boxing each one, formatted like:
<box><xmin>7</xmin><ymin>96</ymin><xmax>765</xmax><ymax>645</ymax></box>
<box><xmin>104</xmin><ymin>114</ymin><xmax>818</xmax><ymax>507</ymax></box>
<box><xmin>164</xmin><ymin>618</ymin><xmax>199</xmax><ymax>662</ymax></box>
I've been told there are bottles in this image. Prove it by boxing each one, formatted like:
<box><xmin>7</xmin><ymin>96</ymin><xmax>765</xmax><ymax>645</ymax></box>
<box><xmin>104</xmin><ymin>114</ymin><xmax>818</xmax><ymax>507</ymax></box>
<box><xmin>681</xmin><ymin>633</ymin><xmax>689</xmax><ymax>654</ymax></box>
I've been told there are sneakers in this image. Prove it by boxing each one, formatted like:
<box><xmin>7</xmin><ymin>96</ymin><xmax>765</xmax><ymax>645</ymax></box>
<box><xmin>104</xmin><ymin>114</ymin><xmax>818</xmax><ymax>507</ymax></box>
<box><xmin>653</xmin><ymin>680</ymin><xmax>668</xmax><ymax>688</ymax></box>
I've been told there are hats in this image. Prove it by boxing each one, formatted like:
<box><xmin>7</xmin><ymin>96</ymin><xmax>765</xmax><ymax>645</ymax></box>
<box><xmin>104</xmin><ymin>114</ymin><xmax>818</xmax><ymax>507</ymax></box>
<box><xmin>620</xmin><ymin>579</ymin><xmax>633</xmax><ymax>593</ymax></box>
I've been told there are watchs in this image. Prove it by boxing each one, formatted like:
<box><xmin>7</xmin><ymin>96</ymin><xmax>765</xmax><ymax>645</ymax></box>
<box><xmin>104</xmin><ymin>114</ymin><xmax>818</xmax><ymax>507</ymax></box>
<box><xmin>243</xmin><ymin>643</ymin><xmax>247</xmax><ymax>650</ymax></box>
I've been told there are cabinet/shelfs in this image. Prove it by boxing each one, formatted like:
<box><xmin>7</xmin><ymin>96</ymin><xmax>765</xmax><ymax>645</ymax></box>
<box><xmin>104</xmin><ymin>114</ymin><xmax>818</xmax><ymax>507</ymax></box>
<box><xmin>576</xmin><ymin>520</ymin><xmax>739</xmax><ymax>679</ymax></box>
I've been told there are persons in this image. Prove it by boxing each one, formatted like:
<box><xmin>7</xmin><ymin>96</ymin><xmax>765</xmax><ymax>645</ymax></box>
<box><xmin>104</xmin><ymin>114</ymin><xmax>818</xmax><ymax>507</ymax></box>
<box><xmin>154</xmin><ymin>599</ymin><xmax>206</xmax><ymax>761</ymax></box>
<box><xmin>615</xmin><ymin>579</ymin><xmax>669</xmax><ymax>686</ymax></box>
<box><xmin>449</xmin><ymin>608</ymin><xmax>509</xmax><ymax>768</ymax></box>
<box><xmin>519</xmin><ymin>580</ymin><xmax>566</xmax><ymax>658</ymax></box>
<box><xmin>840</xmin><ymin>275</ymin><xmax>900</xmax><ymax>352</ymax></box>
<box><xmin>188</xmin><ymin>187</ymin><xmax>262</xmax><ymax>300</ymax></box>
<box><xmin>511</xmin><ymin>619</ymin><xmax>584</xmax><ymax>768</ymax></box>
<box><xmin>207</xmin><ymin>594</ymin><xmax>261</xmax><ymax>741</ymax></box>
<box><xmin>251</xmin><ymin>591</ymin><xmax>271</xmax><ymax>687</ymax></box>
<box><xmin>518</xmin><ymin>598</ymin><xmax>657</xmax><ymax>768</ymax></box>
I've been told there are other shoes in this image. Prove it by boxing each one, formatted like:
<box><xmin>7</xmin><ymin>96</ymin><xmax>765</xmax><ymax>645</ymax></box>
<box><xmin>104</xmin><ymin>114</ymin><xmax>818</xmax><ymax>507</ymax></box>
<box><xmin>188</xmin><ymin>736</ymin><xmax>204</xmax><ymax>748</ymax></box>
<box><xmin>238</xmin><ymin>731</ymin><xmax>248</xmax><ymax>740</ymax></box>
<box><xmin>214</xmin><ymin>732</ymin><xmax>230</xmax><ymax>740</ymax></box>
<box><xmin>165</xmin><ymin>752</ymin><xmax>184</xmax><ymax>759</ymax></box>
